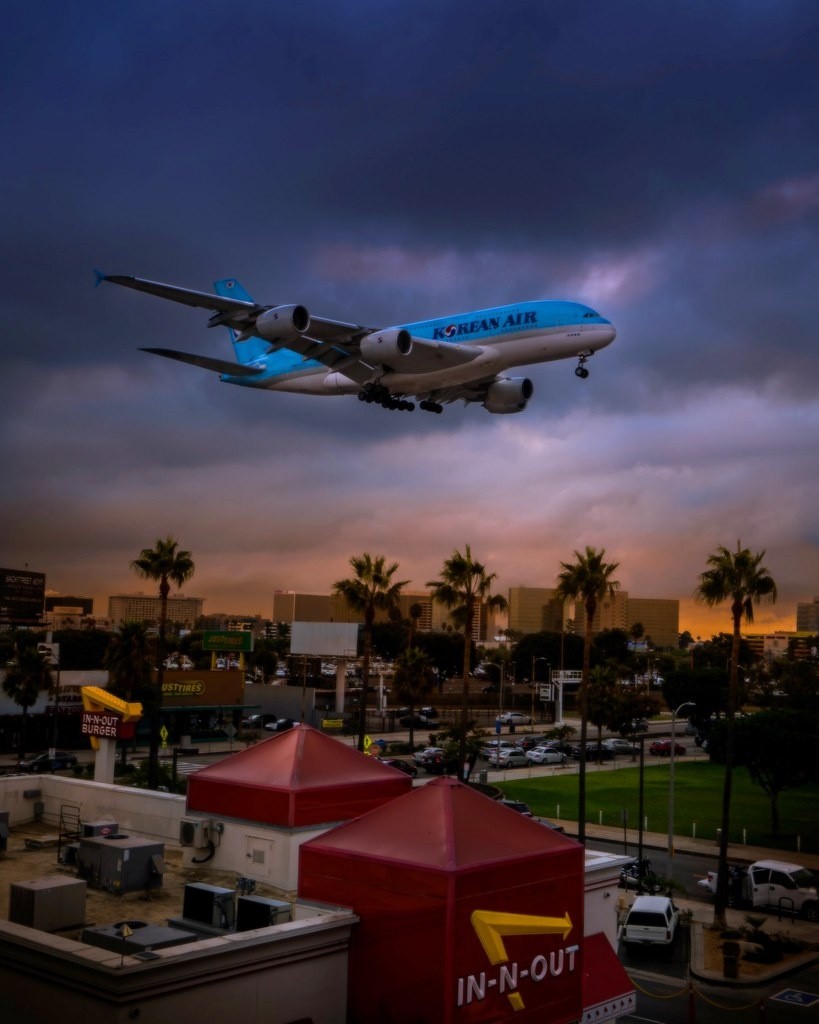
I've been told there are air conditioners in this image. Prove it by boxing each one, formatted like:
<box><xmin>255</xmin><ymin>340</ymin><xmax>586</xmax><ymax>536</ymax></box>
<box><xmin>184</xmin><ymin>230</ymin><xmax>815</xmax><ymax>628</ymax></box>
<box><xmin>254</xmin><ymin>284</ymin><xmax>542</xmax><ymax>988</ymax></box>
<box><xmin>179</xmin><ymin>816</ymin><xmax>211</xmax><ymax>849</ymax></box>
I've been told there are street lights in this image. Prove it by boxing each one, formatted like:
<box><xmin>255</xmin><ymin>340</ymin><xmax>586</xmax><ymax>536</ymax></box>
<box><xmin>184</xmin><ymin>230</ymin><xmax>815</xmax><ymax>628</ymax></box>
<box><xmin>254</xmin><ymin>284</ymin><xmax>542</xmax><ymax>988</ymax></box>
<box><xmin>481</xmin><ymin>659</ymin><xmax>504</xmax><ymax>772</ymax></box>
<box><xmin>531</xmin><ymin>655</ymin><xmax>548</xmax><ymax>734</ymax></box>
<box><xmin>666</xmin><ymin>702</ymin><xmax>698</xmax><ymax>888</ymax></box>
<box><xmin>630</xmin><ymin>733</ymin><xmax>655</xmax><ymax>897</ymax></box>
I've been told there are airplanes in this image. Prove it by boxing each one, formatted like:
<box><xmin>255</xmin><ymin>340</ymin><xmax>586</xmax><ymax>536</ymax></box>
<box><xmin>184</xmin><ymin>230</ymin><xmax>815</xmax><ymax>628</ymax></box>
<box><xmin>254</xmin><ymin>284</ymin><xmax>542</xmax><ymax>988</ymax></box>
<box><xmin>92</xmin><ymin>267</ymin><xmax>618</xmax><ymax>413</ymax></box>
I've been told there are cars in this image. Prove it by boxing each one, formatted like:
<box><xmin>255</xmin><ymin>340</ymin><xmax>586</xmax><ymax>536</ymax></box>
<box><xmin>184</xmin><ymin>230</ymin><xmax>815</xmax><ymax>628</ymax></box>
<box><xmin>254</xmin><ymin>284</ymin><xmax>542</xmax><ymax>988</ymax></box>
<box><xmin>497</xmin><ymin>799</ymin><xmax>666</xmax><ymax>894</ymax></box>
<box><xmin>379</xmin><ymin>747</ymin><xmax>460</xmax><ymax>778</ymax></box>
<box><xmin>571</xmin><ymin>737</ymin><xmax>687</xmax><ymax>761</ymax></box>
<box><xmin>496</xmin><ymin>712</ymin><xmax>537</xmax><ymax>725</ymax></box>
<box><xmin>489</xmin><ymin>750</ymin><xmax>534</xmax><ymax>768</ymax></box>
<box><xmin>18</xmin><ymin>748</ymin><xmax>77</xmax><ymax>773</ymax></box>
<box><xmin>480</xmin><ymin>740</ymin><xmax>525</xmax><ymax>758</ymax></box>
<box><xmin>242</xmin><ymin>713</ymin><xmax>300</xmax><ymax>738</ymax></box>
<box><xmin>527</xmin><ymin>746</ymin><xmax>568</xmax><ymax>766</ymax></box>
<box><xmin>419</xmin><ymin>707</ymin><xmax>438</xmax><ymax>718</ymax></box>
<box><xmin>400</xmin><ymin>715</ymin><xmax>439</xmax><ymax>730</ymax></box>
<box><xmin>621</xmin><ymin>895</ymin><xmax>680</xmax><ymax>947</ymax></box>
<box><xmin>514</xmin><ymin>734</ymin><xmax>574</xmax><ymax>756</ymax></box>
<box><xmin>390</xmin><ymin>707</ymin><xmax>410</xmax><ymax>717</ymax></box>
<box><xmin>164</xmin><ymin>654</ymin><xmax>512</xmax><ymax>694</ymax></box>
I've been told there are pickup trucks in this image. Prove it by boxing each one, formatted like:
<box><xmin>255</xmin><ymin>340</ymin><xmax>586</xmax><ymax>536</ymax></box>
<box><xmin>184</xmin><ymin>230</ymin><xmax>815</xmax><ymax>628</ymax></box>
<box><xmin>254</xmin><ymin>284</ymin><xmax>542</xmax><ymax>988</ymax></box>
<box><xmin>699</xmin><ymin>860</ymin><xmax>819</xmax><ymax>922</ymax></box>
